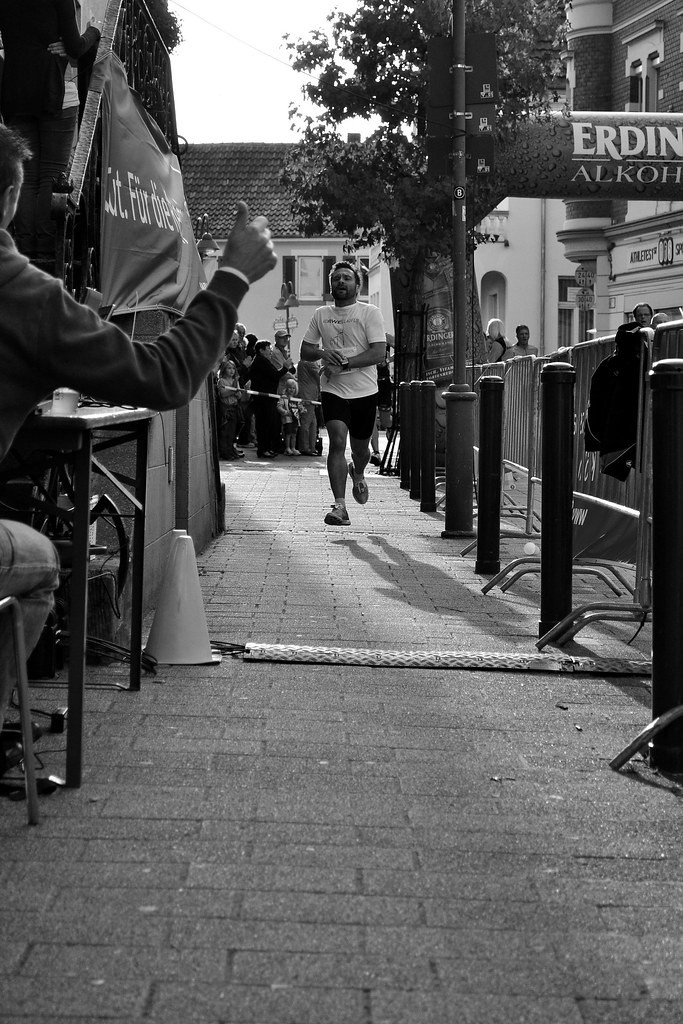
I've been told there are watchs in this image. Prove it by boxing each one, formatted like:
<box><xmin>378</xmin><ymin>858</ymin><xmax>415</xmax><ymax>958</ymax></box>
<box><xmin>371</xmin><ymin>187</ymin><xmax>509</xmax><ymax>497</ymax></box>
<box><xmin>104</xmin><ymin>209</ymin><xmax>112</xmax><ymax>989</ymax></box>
<box><xmin>385</xmin><ymin>357</ymin><xmax>390</xmax><ymax>364</ymax></box>
<box><xmin>341</xmin><ymin>356</ymin><xmax>349</xmax><ymax>370</ymax></box>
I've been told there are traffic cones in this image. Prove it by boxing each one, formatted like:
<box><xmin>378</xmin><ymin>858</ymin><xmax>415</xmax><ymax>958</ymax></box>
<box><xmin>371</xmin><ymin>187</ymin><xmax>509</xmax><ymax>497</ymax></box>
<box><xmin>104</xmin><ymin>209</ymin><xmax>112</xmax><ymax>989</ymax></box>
<box><xmin>140</xmin><ymin>529</ymin><xmax>222</xmax><ymax>665</ymax></box>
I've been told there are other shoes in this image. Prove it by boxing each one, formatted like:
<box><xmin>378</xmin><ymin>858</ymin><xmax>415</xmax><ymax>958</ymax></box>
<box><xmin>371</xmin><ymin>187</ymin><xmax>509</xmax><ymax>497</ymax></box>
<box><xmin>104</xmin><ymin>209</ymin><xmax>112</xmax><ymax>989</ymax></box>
<box><xmin>0</xmin><ymin>741</ymin><xmax>23</xmax><ymax>771</ymax></box>
<box><xmin>258</xmin><ymin>452</ymin><xmax>275</xmax><ymax>458</ymax></box>
<box><xmin>371</xmin><ymin>451</ymin><xmax>383</xmax><ymax>467</ymax></box>
<box><xmin>292</xmin><ymin>448</ymin><xmax>301</xmax><ymax>456</ymax></box>
<box><xmin>270</xmin><ymin>450</ymin><xmax>278</xmax><ymax>457</ymax></box>
<box><xmin>283</xmin><ymin>449</ymin><xmax>296</xmax><ymax>457</ymax></box>
<box><xmin>228</xmin><ymin>442</ymin><xmax>258</xmax><ymax>461</ymax></box>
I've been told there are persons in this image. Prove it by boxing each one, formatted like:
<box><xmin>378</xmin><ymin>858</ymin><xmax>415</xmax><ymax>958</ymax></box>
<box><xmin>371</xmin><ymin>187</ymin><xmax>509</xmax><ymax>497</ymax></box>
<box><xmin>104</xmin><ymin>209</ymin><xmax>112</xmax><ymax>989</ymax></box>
<box><xmin>631</xmin><ymin>302</ymin><xmax>670</xmax><ymax>328</ymax></box>
<box><xmin>300</xmin><ymin>261</ymin><xmax>387</xmax><ymax>525</ymax></box>
<box><xmin>484</xmin><ymin>319</ymin><xmax>538</xmax><ymax>362</ymax></box>
<box><xmin>0</xmin><ymin>0</ymin><xmax>103</xmax><ymax>261</ymax></box>
<box><xmin>211</xmin><ymin>324</ymin><xmax>395</xmax><ymax>465</ymax></box>
<box><xmin>0</xmin><ymin>126</ymin><xmax>279</xmax><ymax>777</ymax></box>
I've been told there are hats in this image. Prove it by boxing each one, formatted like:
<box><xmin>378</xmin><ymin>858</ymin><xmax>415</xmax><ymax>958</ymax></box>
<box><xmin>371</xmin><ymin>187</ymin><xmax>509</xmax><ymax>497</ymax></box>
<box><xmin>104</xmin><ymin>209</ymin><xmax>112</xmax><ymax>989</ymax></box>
<box><xmin>274</xmin><ymin>329</ymin><xmax>293</xmax><ymax>338</ymax></box>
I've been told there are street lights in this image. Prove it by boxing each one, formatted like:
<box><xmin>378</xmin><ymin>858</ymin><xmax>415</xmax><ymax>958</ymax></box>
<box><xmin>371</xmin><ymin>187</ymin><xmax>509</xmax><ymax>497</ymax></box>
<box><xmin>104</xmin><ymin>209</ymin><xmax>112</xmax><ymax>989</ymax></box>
<box><xmin>274</xmin><ymin>281</ymin><xmax>299</xmax><ymax>358</ymax></box>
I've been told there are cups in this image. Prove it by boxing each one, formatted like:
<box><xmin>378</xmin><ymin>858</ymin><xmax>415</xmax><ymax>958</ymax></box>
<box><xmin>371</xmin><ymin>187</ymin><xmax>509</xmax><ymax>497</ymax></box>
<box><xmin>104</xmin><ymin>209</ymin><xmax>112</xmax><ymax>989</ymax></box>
<box><xmin>52</xmin><ymin>388</ymin><xmax>78</xmax><ymax>415</ymax></box>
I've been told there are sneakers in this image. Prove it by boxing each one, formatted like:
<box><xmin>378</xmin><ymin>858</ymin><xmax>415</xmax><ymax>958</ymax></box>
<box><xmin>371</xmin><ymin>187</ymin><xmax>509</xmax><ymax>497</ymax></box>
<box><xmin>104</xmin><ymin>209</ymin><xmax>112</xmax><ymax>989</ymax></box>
<box><xmin>348</xmin><ymin>462</ymin><xmax>369</xmax><ymax>505</ymax></box>
<box><xmin>324</xmin><ymin>502</ymin><xmax>352</xmax><ymax>526</ymax></box>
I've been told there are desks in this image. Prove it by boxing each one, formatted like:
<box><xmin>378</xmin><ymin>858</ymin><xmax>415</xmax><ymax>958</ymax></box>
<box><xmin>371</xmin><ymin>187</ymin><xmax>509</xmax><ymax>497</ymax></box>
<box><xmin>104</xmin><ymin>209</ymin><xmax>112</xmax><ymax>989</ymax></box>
<box><xmin>0</xmin><ymin>402</ymin><xmax>155</xmax><ymax>790</ymax></box>
